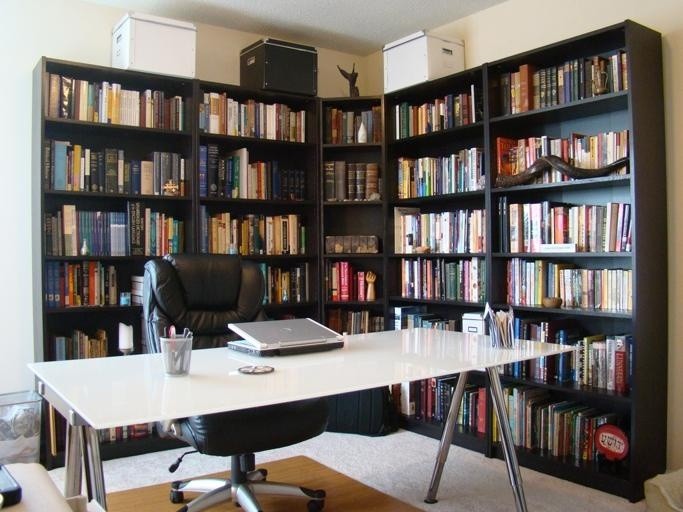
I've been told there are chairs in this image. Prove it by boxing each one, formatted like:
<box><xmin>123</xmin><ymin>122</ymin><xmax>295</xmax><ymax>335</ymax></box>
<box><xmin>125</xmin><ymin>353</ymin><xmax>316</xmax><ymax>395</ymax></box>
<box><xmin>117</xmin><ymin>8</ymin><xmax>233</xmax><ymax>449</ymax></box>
<box><xmin>142</xmin><ymin>252</ymin><xmax>327</xmax><ymax>512</ymax></box>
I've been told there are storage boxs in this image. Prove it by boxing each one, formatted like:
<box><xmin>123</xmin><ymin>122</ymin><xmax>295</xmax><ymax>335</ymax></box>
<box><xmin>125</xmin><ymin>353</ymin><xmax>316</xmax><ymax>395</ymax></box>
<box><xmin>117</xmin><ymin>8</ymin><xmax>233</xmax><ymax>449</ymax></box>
<box><xmin>240</xmin><ymin>37</ymin><xmax>319</xmax><ymax>95</ymax></box>
<box><xmin>382</xmin><ymin>30</ymin><xmax>466</xmax><ymax>95</ymax></box>
<box><xmin>110</xmin><ymin>12</ymin><xmax>196</xmax><ymax>80</ymax></box>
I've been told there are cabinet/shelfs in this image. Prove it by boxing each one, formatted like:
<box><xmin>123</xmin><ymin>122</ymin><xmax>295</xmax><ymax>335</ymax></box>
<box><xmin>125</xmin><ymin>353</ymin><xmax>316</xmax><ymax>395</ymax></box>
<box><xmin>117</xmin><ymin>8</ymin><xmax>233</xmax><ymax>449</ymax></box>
<box><xmin>385</xmin><ymin>62</ymin><xmax>487</xmax><ymax>457</ymax></box>
<box><xmin>484</xmin><ymin>20</ymin><xmax>667</xmax><ymax>504</ymax></box>
<box><xmin>30</xmin><ymin>56</ymin><xmax>197</xmax><ymax>363</ymax></box>
<box><xmin>319</xmin><ymin>94</ymin><xmax>388</xmax><ymax>339</ymax></box>
<box><xmin>197</xmin><ymin>78</ymin><xmax>323</xmax><ymax>330</ymax></box>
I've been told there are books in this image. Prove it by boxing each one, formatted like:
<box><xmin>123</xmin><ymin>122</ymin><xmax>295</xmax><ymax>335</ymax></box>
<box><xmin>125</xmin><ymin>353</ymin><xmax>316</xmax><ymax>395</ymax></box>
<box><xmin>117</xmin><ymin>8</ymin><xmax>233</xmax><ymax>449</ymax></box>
<box><xmin>326</xmin><ymin>261</ymin><xmax>367</xmax><ymax>301</ymax></box>
<box><xmin>324</xmin><ymin>161</ymin><xmax>382</xmax><ymax>201</ymax></box>
<box><xmin>396</xmin><ymin>85</ymin><xmax>484</xmax><ymax>141</ymax></box>
<box><xmin>497</xmin><ymin>130</ymin><xmax>628</xmax><ymax>186</ymax></box>
<box><xmin>329</xmin><ymin>309</ymin><xmax>384</xmax><ymax>335</ymax></box>
<box><xmin>398</xmin><ymin>148</ymin><xmax>484</xmax><ymax>198</ymax></box>
<box><xmin>44</xmin><ymin>138</ymin><xmax>185</xmax><ymax>197</ymax></box>
<box><xmin>45</xmin><ymin>73</ymin><xmax>184</xmax><ymax>131</ymax></box>
<box><xmin>506</xmin><ymin>258</ymin><xmax>632</xmax><ymax>310</ymax></box>
<box><xmin>45</xmin><ymin>202</ymin><xmax>184</xmax><ymax>256</ymax></box>
<box><xmin>499</xmin><ymin>51</ymin><xmax>626</xmax><ymax>115</ymax></box>
<box><xmin>49</xmin><ymin>402</ymin><xmax>152</xmax><ymax>456</ymax></box>
<box><xmin>257</xmin><ymin>262</ymin><xmax>309</xmax><ymax>303</ymax></box>
<box><xmin>201</xmin><ymin>205</ymin><xmax>305</xmax><ymax>254</ymax></box>
<box><xmin>200</xmin><ymin>93</ymin><xmax>305</xmax><ymax>142</ymax></box>
<box><xmin>498</xmin><ymin>198</ymin><xmax>631</xmax><ymax>252</ymax></box>
<box><xmin>46</xmin><ymin>261</ymin><xmax>145</xmax><ymax>307</ymax></box>
<box><xmin>402</xmin><ymin>258</ymin><xmax>486</xmax><ymax>303</ymax></box>
<box><xmin>48</xmin><ymin>329</ymin><xmax>107</xmax><ymax>364</ymax></box>
<box><xmin>394</xmin><ymin>206</ymin><xmax>486</xmax><ymax>253</ymax></box>
<box><xmin>324</xmin><ymin>107</ymin><xmax>382</xmax><ymax>144</ymax></box>
<box><xmin>389</xmin><ymin>375</ymin><xmax>488</xmax><ymax>433</ymax></box>
<box><xmin>498</xmin><ymin>316</ymin><xmax>633</xmax><ymax>392</ymax></box>
<box><xmin>395</xmin><ymin>306</ymin><xmax>461</xmax><ymax>333</ymax></box>
<box><xmin>493</xmin><ymin>385</ymin><xmax>616</xmax><ymax>462</ymax></box>
<box><xmin>200</xmin><ymin>145</ymin><xmax>305</xmax><ymax>201</ymax></box>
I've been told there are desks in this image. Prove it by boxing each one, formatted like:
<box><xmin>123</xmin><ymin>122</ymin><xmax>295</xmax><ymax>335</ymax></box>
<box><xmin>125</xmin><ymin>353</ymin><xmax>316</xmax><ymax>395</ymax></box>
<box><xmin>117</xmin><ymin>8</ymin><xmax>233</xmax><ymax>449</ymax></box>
<box><xmin>29</xmin><ymin>328</ymin><xmax>578</xmax><ymax>512</ymax></box>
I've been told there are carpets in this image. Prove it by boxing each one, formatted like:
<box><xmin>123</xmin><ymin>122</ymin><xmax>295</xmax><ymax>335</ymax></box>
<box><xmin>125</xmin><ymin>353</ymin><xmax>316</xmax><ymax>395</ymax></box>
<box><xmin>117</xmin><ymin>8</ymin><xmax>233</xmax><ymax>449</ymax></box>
<box><xmin>106</xmin><ymin>455</ymin><xmax>429</xmax><ymax>512</ymax></box>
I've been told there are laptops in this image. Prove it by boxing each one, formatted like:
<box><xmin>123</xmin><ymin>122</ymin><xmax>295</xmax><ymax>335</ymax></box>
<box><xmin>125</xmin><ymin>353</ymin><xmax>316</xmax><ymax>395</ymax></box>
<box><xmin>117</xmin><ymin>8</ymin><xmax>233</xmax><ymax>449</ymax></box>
<box><xmin>228</xmin><ymin>318</ymin><xmax>344</xmax><ymax>357</ymax></box>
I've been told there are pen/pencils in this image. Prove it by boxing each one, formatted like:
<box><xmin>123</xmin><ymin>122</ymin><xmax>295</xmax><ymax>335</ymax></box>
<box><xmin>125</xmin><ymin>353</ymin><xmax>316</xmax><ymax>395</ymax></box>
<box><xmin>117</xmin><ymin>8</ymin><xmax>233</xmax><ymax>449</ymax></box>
<box><xmin>160</xmin><ymin>324</ymin><xmax>193</xmax><ymax>372</ymax></box>
